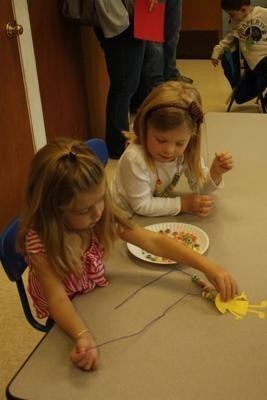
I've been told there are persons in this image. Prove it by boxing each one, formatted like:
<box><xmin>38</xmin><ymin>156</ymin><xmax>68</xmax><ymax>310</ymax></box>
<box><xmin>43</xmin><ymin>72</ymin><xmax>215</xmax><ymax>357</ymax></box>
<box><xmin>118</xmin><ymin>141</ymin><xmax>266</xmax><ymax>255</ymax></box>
<box><xmin>109</xmin><ymin>81</ymin><xmax>235</xmax><ymax>218</ymax></box>
<box><xmin>91</xmin><ymin>0</ymin><xmax>151</xmax><ymax>161</ymax></box>
<box><xmin>18</xmin><ymin>139</ymin><xmax>239</xmax><ymax>373</ymax></box>
<box><xmin>134</xmin><ymin>0</ymin><xmax>193</xmax><ymax>116</ymax></box>
<box><xmin>212</xmin><ymin>1</ymin><xmax>266</xmax><ymax>112</ymax></box>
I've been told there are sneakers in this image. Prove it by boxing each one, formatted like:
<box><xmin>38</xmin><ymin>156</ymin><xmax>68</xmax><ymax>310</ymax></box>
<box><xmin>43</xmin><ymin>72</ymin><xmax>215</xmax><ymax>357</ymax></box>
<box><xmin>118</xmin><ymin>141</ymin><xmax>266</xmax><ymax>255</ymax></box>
<box><xmin>106</xmin><ymin>139</ymin><xmax>126</xmax><ymax>159</ymax></box>
<box><xmin>177</xmin><ymin>76</ymin><xmax>193</xmax><ymax>84</ymax></box>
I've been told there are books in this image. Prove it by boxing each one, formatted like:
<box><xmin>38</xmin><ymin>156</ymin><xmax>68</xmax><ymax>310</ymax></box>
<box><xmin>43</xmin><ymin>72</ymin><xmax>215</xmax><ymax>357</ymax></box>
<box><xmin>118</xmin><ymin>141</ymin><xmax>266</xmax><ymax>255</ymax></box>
<box><xmin>133</xmin><ymin>0</ymin><xmax>166</xmax><ymax>43</ymax></box>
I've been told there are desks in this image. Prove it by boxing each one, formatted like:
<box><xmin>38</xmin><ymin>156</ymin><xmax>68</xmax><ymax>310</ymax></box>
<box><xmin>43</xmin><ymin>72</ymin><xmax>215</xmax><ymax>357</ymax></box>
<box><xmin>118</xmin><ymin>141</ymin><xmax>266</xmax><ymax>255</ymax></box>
<box><xmin>6</xmin><ymin>112</ymin><xmax>267</xmax><ymax>400</ymax></box>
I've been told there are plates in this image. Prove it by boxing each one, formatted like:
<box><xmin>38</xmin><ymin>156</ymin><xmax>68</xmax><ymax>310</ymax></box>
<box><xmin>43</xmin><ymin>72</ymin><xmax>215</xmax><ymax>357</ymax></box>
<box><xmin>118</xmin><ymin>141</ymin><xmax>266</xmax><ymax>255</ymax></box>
<box><xmin>126</xmin><ymin>222</ymin><xmax>210</xmax><ymax>265</ymax></box>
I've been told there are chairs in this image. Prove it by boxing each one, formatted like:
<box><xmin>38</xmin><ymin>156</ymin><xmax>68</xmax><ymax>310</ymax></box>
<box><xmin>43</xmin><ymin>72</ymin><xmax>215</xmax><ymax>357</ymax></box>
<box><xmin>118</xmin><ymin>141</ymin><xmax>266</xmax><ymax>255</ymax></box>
<box><xmin>221</xmin><ymin>51</ymin><xmax>267</xmax><ymax>113</ymax></box>
<box><xmin>0</xmin><ymin>213</ymin><xmax>56</xmax><ymax>333</ymax></box>
<box><xmin>83</xmin><ymin>138</ymin><xmax>108</xmax><ymax>168</ymax></box>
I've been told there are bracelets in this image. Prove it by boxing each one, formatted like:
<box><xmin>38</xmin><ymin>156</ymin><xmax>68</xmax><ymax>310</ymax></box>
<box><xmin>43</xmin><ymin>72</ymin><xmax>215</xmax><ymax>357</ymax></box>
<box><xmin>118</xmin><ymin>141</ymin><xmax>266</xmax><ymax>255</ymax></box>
<box><xmin>73</xmin><ymin>329</ymin><xmax>91</xmax><ymax>343</ymax></box>
